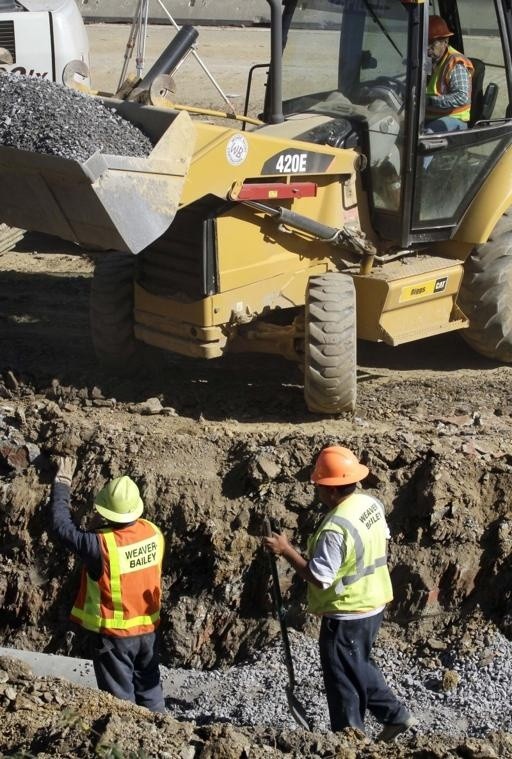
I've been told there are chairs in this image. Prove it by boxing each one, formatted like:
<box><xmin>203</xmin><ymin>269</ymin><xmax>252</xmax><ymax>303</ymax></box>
<box><xmin>467</xmin><ymin>57</ymin><xmax>499</xmax><ymax>129</ymax></box>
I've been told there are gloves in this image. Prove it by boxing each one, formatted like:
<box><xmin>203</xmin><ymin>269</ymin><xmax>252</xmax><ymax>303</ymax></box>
<box><xmin>54</xmin><ymin>456</ymin><xmax>77</xmax><ymax>487</ymax></box>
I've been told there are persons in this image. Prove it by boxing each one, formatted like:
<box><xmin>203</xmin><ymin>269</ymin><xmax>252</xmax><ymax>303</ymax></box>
<box><xmin>265</xmin><ymin>445</ymin><xmax>416</xmax><ymax>743</ymax></box>
<box><xmin>420</xmin><ymin>15</ymin><xmax>475</xmax><ymax>137</ymax></box>
<box><xmin>49</xmin><ymin>455</ymin><xmax>167</xmax><ymax>720</ymax></box>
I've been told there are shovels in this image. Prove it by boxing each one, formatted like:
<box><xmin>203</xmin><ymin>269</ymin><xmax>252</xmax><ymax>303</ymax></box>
<box><xmin>263</xmin><ymin>518</ymin><xmax>311</xmax><ymax>732</ymax></box>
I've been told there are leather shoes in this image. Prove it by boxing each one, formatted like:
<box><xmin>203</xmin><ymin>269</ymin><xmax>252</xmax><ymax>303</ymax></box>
<box><xmin>378</xmin><ymin>714</ymin><xmax>416</xmax><ymax>744</ymax></box>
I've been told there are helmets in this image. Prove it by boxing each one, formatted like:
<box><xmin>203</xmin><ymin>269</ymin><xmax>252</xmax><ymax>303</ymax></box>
<box><xmin>310</xmin><ymin>445</ymin><xmax>370</xmax><ymax>486</ymax></box>
<box><xmin>93</xmin><ymin>475</ymin><xmax>144</xmax><ymax>523</ymax></box>
<box><xmin>428</xmin><ymin>16</ymin><xmax>455</xmax><ymax>40</ymax></box>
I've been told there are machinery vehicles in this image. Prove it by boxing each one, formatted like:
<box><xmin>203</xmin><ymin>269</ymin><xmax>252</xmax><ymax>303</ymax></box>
<box><xmin>0</xmin><ymin>0</ymin><xmax>510</xmax><ymax>413</ymax></box>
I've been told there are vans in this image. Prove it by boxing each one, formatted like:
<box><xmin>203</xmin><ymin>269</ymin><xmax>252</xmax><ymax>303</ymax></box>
<box><xmin>0</xmin><ymin>2</ymin><xmax>90</xmax><ymax>90</ymax></box>
